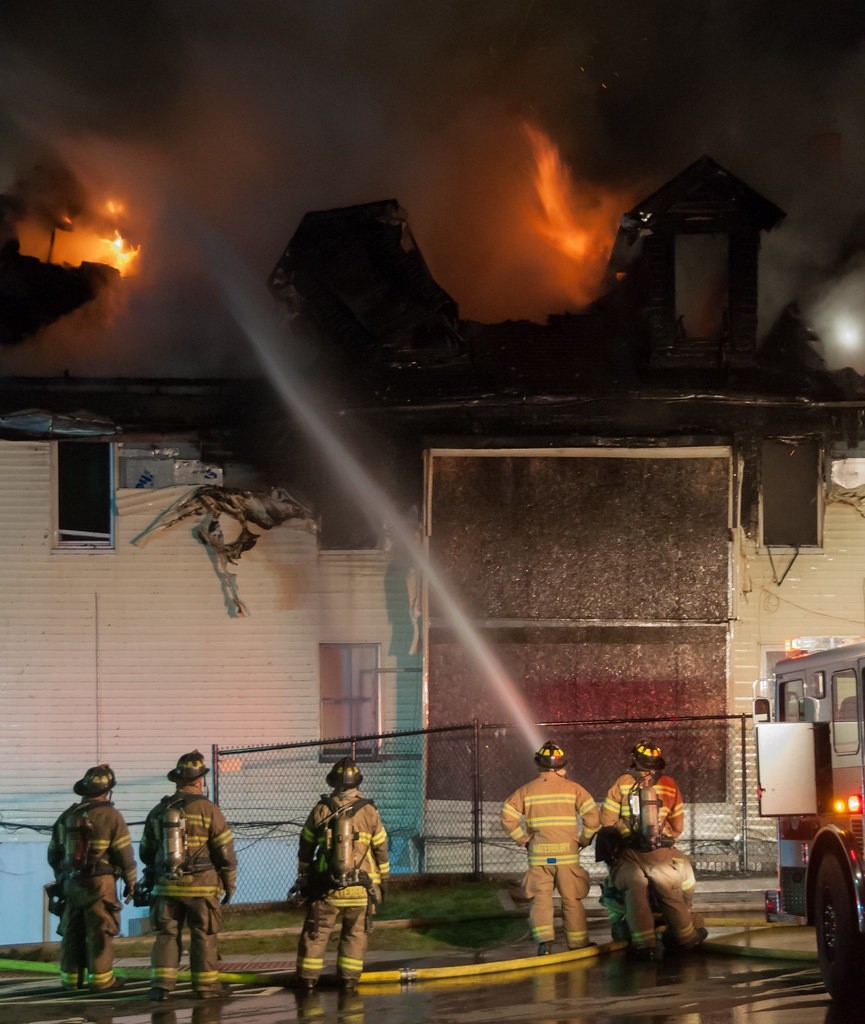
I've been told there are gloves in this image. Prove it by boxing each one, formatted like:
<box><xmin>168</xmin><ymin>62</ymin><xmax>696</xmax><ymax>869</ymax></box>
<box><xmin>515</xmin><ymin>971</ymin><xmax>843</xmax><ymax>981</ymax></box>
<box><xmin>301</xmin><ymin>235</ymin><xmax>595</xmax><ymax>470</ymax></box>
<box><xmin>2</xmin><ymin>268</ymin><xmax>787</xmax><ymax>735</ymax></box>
<box><xmin>122</xmin><ymin>879</ymin><xmax>136</xmax><ymax>904</ymax></box>
<box><xmin>220</xmin><ymin>888</ymin><xmax>235</xmax><ymax>910</ymax></box>
<box><xmin>290</xmin><ymin>877</ymin><xmax>307</xmax><ymax>897</ymax></box>
<box><xmin>379</xmin><ymin>879</ymin><xmax>387</xmax><ymax>904</ymax></box>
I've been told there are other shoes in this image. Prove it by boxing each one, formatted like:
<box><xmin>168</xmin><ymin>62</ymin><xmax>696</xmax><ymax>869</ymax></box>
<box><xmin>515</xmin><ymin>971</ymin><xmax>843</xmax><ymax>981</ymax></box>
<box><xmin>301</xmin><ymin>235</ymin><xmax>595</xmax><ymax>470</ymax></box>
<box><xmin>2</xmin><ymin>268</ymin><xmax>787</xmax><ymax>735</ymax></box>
<box><xmin>92</xmin><ymin>977</ymin><xmax>125</xmax><ymax>994</ymax></box>
<box><xmin>146</xmin><ymin>987</ymin><xmax>170</xmax><ymax>1001</ymax></box>
<box><xmin>64</xmin><ymin>982</ymin><xmax>87</xmax><ymax>989</ymax></box>
<box><xmin>194</xmin><ymin>985</ymin><xmax>234</xmax><ymax>999</ymax></box>
<box><xmin>537</xmin><ymin>941</ymin><xmax>554</xmax><ymax>955</ymax></box>
<box><xmin>627</xmin><ymin>946</ymin><xmax>657</xmax><ymax>958</ymax></box>
<box><xmin>343</xmin><ymin>986</ymin><xmax>359</xmax><ymax>997</ymax></box>
<box><xmin>301</xmin><ymin>979</ymin><xmax>314</xmax><ymax>992</ymax></box>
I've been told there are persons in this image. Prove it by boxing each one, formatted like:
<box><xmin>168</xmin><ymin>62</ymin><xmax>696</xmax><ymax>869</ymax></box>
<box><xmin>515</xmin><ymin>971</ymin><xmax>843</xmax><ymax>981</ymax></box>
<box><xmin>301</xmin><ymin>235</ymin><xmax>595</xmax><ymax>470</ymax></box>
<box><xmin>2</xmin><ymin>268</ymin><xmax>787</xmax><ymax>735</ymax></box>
<box><xmin>501</xmin><ymin>742</ymin><xmax>600</xmax><ymax>956</ymax></box>
<box><xmin>600</xmin><ymin>738</ymin><xmax>708</xmax><ymax>965</ymax></box>
<box><xmin>287</xmin><ymin>757</ymin><xmax>390</xmax><ymax>996</ymax></box>
<box><xmin>47</xmin><ymin>764</ymin><xmax>138</xmax><ymax>993</ymax></box>
<box><xmin>139</xmin><ymin>750</ymin><xmax>237</xmax><ymax>1000</ymax></box>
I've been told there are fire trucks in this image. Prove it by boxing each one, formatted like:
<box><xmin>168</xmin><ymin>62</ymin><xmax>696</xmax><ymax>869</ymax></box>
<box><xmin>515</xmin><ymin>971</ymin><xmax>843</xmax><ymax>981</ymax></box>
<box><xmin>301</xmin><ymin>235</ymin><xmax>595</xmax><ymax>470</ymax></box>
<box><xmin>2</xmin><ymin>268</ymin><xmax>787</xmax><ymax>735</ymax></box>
<box><xmin>753</xmin><ymin>636</ymin><xmax>865</xmax><ymax>1015</ymax></box>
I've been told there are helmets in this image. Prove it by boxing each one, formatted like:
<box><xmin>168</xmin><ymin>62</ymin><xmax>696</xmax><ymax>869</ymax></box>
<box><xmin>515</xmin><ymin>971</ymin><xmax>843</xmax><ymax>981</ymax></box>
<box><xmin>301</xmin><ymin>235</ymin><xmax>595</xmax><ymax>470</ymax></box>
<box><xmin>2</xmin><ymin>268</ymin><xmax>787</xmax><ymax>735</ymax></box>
<box><xmin>533</xmin><ymin>741</ymin><xmax>569</xmax><ymax>771</ymax></box>
<box><xmin>73</xmin><ymin>764</ymin><xmax>116</xmax><ymax>798</ymax></box>
<box><xmin>325</xmin><ymin>757</ymin><xmax>364</xmax><ymax>789</ymax></box>
<box><xmin>632</xmin><ymin>740</ymin><xmax>668</xmax><ymax>770</ymax></box>
<box><xmin>167</xmin><ymin>750</ymin><xmax>211</xmax><ymax>781</ymax></box>
